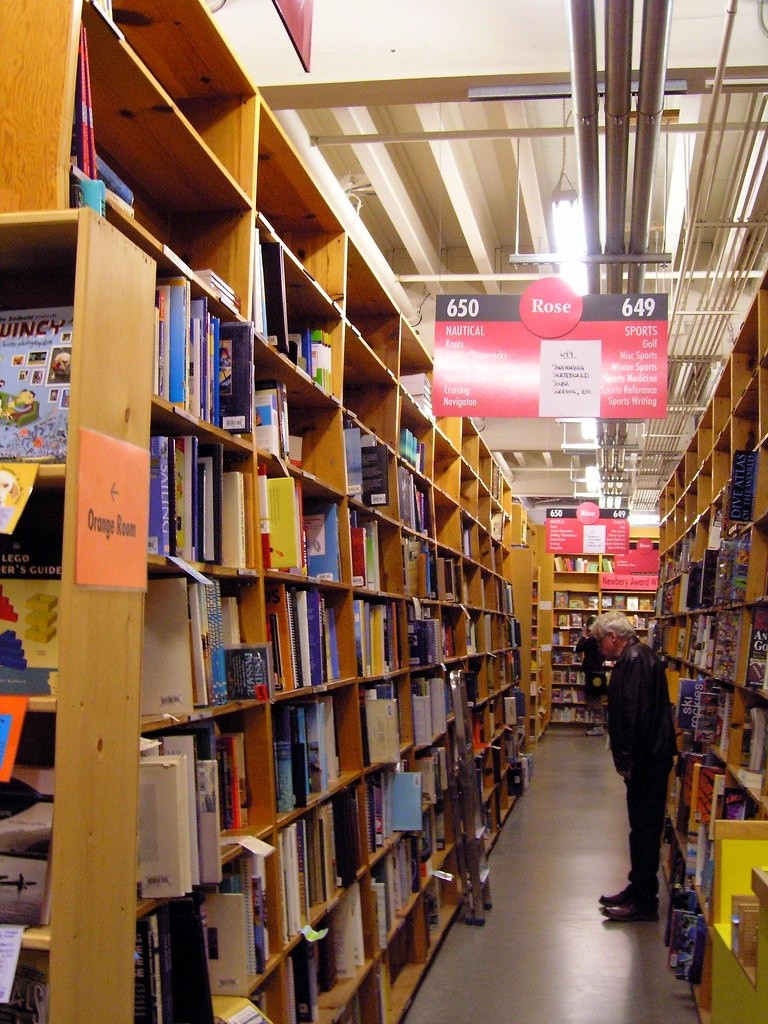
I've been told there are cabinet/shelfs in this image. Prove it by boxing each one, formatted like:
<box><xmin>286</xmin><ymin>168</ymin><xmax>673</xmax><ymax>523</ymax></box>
<box><xmin>649</xmin><ymin>267</ymin><xmax>768</xmax><ymax>1024</ymax></box>
<box><xmin>553</xmin><ymin>527</ymin><xmax>659</xmax><ymax>729</ymax></box>
<box><xmin>0</xmin><ymin>0</ymin><xmax>554</xmax><ymax>1024</ymax></box>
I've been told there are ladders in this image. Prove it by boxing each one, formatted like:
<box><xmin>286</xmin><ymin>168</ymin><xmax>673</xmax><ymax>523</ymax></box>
<box><xmin>449</xmin><ymin>668</ymin><xmax>492</xmax><ymax>925</ymax></box>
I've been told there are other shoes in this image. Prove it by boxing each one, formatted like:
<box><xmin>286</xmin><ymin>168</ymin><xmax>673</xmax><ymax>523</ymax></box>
<box><xmin>585</xmin><ymin>727</ymin><xmax>603</xmax><ymax>736</ymax></box>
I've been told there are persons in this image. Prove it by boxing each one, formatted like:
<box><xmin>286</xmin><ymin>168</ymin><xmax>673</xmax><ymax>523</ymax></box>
<box><xmin>588</xmin><ymin>610</ymin><xmax>679</xmax><ymax>921</ymax></box>
<box><xmin>574</xmin><ymin>617</ymin><xmax>608</xmax><ymax>736</ymax></box>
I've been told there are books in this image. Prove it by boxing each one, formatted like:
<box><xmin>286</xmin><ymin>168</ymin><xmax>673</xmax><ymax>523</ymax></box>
<box><xmin>0</xmin><ymin>0</ymin><xmax>768</xmax><ymax>1024</ymax></box>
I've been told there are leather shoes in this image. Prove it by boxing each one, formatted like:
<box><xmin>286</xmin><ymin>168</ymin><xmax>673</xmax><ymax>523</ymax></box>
<box><xmin>603</xmin><ymin>898</ymin><xmax>659</xmax><ymax>921</ymax></box>
<box><xmin>599</xmin><ymin>884</ymin><xmax>632</xmax><ymax>906</ymax></box>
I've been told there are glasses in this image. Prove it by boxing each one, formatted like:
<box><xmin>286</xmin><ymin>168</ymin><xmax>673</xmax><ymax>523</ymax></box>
<box><xmin>595</xmin><ymin>631</ymin><xmax>609</xmax><ymax>647</ymax></box>
